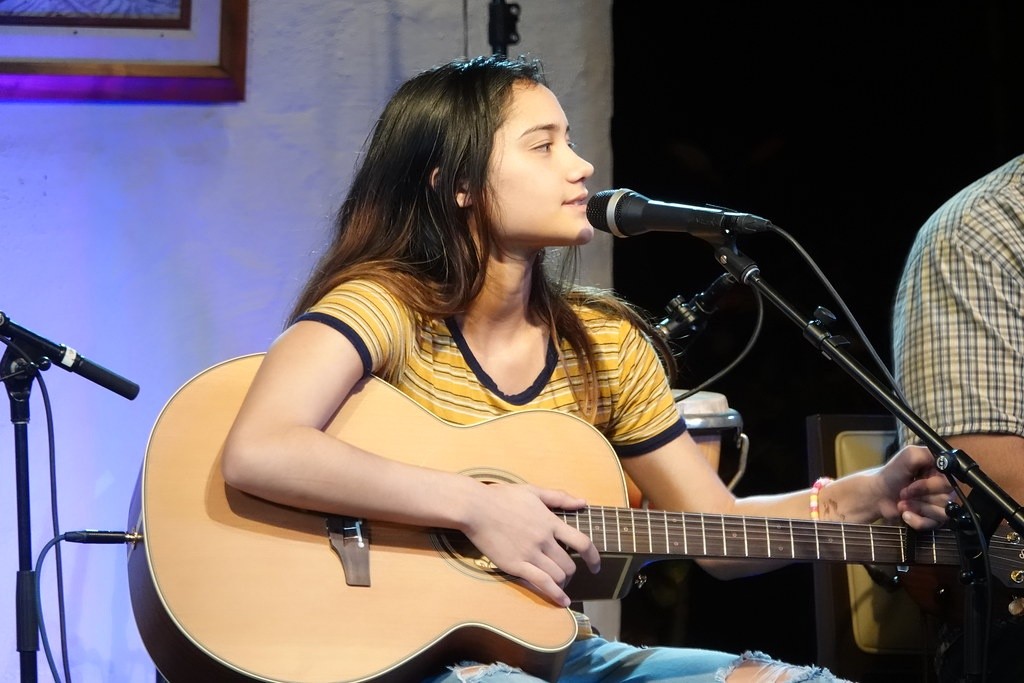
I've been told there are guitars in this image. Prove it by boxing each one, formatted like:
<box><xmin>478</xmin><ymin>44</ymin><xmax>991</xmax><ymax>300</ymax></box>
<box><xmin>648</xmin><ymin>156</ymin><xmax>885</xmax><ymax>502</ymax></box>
<box><xmin>126</xmin><ymin>350</ymin><xmax>1024</xmax><ymax>682</ymax></box>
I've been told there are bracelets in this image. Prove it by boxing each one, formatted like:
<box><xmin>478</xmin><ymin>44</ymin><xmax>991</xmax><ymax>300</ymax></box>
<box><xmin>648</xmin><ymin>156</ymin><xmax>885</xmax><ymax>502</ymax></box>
<box><xmin>809</xmin><ymin>476</ymin><xmax>836</xmax><ymax>521</ymax></box>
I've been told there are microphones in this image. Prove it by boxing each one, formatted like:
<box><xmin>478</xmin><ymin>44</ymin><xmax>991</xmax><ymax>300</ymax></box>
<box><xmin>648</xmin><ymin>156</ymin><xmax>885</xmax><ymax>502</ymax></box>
<box><xmin>586</xmin><ymin>188</ymin><xmax>772</xmax><ymax>238</ymax></box>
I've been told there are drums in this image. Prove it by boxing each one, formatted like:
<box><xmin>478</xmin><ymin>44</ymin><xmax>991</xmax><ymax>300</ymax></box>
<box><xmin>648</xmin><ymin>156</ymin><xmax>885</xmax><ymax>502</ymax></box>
<box><xmin>622</xmin><ymin>386</ymin><xmax>744</xmax><ymax>511</ymax></box>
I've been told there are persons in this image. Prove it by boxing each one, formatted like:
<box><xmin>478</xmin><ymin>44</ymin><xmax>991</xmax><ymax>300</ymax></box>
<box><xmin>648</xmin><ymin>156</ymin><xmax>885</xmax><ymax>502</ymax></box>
<box><xmin>892</xmin><ymin>151</ymin><xmax>1024</xmax><ymax>683</ymax></box>
<box><xmin>219</xmin><ymin>53</ymin><xmax>952</xmax><ymax>683</ymax></box>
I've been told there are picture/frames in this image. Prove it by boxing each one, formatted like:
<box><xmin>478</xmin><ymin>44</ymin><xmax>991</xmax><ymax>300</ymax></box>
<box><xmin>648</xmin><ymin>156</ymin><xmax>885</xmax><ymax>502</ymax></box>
<box><xmin>0</xmin><ymin>1</ymin><xmax>256</xmax><ymax>112</ymax></box>
